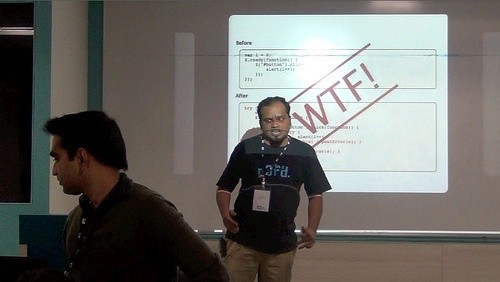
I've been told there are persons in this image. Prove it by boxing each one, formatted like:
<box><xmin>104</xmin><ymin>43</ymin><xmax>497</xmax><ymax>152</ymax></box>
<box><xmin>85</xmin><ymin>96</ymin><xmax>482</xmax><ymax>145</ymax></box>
<box><xmin>43</xmin><ymin>111</ymin><xmax>229</xmax><ymax>282</ymax></box>
<box><xmin>216</xmin><ymin>97</ymin><xmax>331</xmax><ymax>282</ymax></box>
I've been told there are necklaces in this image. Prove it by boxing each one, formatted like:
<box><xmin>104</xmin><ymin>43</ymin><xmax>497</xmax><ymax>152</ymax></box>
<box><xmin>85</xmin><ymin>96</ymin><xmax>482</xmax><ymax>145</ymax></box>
<box><xmin>60</xmin><ymin>211</ymin><xmax>94</xmax><ymax>282</ymax></box>
